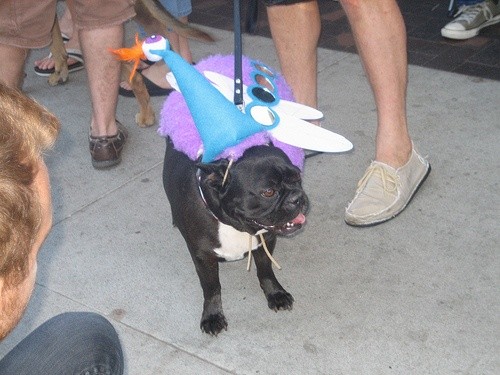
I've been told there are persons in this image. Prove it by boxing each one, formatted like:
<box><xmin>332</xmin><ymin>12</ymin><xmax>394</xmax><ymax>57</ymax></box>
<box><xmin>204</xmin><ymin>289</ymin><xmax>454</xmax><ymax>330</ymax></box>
<box><xmin>441</xmin><ymin>0</ymin><xmax>500</xmax><ymax>39</ymax></box>
<box><xmin>33</xmin><ymin>0</ymin><xmax>191</xmax><ymax>98</ymax></box>
<box><xmin>264</xmin><ymin>0</ymin><xmax>431</xmax><ymax>226</ymax></box>
<box><xmin>0</xmin><ymin>80</ymin><xmax>126</xmax><ymax>375</ymax></box>
<box><xmin>0</xmin><ymin>0</ymin><xmax>137</xmax><ymax>169</ymax></box>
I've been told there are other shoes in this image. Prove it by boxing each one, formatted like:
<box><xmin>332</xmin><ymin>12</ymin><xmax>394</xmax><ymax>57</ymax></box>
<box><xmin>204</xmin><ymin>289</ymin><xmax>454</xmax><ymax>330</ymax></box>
<box><xmin>89</xmin><ymin>119</ymin><xmax>128</xmax><ymax>168</ymax></box>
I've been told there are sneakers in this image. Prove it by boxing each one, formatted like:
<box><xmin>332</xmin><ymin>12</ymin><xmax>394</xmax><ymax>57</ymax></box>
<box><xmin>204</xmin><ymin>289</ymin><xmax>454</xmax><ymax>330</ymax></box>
<box><xmin>304</xmin><ymin>150</ymin><xmax>318</xmax><ymax>157</ymax></box>
<box><xmin>441</xmin><ymin>0</ymin><xmax>500</xmax><ymax>39</ymax></box>
<box><xmin>345</xmin><ymin>141</ymin><xmax>431</xmax><ymax>225</ymax></box>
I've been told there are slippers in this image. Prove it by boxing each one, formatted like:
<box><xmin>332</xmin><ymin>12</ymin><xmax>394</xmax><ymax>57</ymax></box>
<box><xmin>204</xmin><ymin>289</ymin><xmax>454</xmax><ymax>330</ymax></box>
<box><xmin>33</xmin><ymin>48</ymin><xmax>85</xmax><ymax>76</ymax></box>
<box><xmin>130</xmin><ymin>57</ymin><xmax>155</xmax><ymax>71</ymax></box>
<box><xmin>61</xmin><ymin>33</ymin><xmax>70</xmax><ymax>43</ymax></box>
<box><xmin>118</xmin><ymin>61</ymin><xmax>195</xmax><ymax>96</ymax></box>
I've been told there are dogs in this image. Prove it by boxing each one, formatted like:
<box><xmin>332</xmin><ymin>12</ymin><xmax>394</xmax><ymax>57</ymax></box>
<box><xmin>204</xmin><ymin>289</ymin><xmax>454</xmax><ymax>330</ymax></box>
<box><xmin>160</xmin><ymin>133</ymin><xmax>310</xmax><ymax>337</ymax></box>
